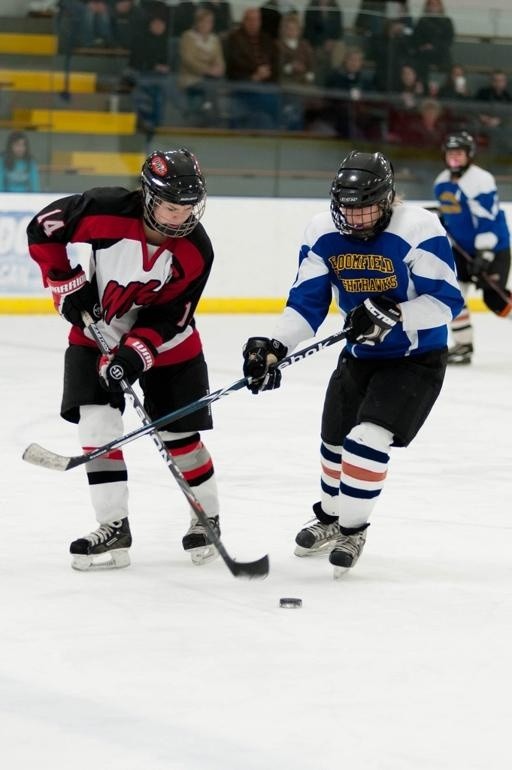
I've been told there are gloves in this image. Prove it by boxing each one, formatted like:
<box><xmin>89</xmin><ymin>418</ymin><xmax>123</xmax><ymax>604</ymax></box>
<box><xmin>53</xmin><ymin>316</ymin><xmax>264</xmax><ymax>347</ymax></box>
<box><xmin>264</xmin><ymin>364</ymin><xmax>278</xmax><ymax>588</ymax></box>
<box><xmin>96</xmin><ymin>332</ymin><xmax>159</xmax><ymax>393</ymax></box>
<box><xmin>243</xmin><ymin>336</ymin><xmax>288</xmax><ymax>394</ymax></box>
<box><xmin>47</xmin><ymin>264</ymin><xmax>101</xmax><ymax>326</ymax></box>
<box><xmin>343</xmin><ymin>296</ymin><xmax>402</xmax><ymax>347</ymax></box>
<box><xmin>468</xmin><ymin>250</ymin><xmax>496</xmax><ymax>278</ymax></box>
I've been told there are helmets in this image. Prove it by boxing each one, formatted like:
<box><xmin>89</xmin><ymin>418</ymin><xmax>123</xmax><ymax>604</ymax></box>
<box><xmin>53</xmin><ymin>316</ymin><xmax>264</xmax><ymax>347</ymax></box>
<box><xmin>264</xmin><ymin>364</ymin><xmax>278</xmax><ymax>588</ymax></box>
<box><xmin>330</xmin><ymin>150</ymin><xmax>395</xmax><ymax>242</ymax></box>
<box><xmin>140</xmin><ymin>148</ymin><xmax>208</xmax><ymax>239</ymax></box>
<box><xmin>439</xmin><ymin>131</ymin><xmax>477</xmax><ymax>178</ymax></box>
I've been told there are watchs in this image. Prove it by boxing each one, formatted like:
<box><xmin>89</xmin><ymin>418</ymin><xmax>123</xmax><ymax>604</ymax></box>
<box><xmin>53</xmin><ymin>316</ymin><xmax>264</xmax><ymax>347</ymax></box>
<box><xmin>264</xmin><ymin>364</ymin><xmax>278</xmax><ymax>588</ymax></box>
<box><xmin>431</xmin><ymin>127</ymin><xmax>512</xmax><ymax>357</ymax></box>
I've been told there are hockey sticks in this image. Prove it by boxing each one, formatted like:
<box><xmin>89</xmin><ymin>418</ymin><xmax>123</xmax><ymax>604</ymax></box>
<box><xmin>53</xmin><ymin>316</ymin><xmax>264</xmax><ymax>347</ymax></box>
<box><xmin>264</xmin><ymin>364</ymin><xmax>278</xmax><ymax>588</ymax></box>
<box><xmin>82</xmin><ymin>313</ymin><xmax>270</xmax><ymax>577</ymax></box>
<box><xmin>23</xmin><ymin>328</ymin><xmax>353</xmax><ymax>470</ymax></box>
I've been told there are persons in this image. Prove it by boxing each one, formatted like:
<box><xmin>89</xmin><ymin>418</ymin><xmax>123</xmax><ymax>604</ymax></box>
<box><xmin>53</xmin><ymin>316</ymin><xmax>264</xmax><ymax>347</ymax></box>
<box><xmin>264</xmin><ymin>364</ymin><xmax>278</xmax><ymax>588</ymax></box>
<box><xmin>23</xmin><ymin>142</ymin><xmax>222</xmax><ymax>555</ymax></box>
<box><xmin>442</xmin><ymin>63</ymin><xmax>512</xmax><ymax>148</ymax></box>
<box><xmin>50</xmin><ymin>1</ymin><xmax>455</xmax><ymax>148</ymax></box>
<box><xmin>0</xmin><ymin>129</ymin><xmax>45</xmax><ymax>193</ymax></box>
<box><xmin>239</xmin><ymin>146</ymin><xmax>469</xmax><ymax>570</ymax></box>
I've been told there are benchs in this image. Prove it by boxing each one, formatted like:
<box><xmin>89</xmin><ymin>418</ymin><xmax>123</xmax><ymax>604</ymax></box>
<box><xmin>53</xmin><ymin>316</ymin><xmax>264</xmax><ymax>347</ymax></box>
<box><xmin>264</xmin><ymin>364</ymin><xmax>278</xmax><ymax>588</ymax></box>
<box><xmin>0</xmin><ymin>2</ymin><xmax>512</xmax><ymax>200</ymax></box>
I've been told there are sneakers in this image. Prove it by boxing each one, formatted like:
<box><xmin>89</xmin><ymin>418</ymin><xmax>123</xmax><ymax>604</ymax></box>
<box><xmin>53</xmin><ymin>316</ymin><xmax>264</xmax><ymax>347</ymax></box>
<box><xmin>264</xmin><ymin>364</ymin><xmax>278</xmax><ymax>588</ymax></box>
<box><xmin>449</xmin><ymin>344</ymin><xmax>472</xmax><ymax>358</ymax></box>
<box><xmin>183</xmin><ymin>514</ymin><xmax>220</xmax><ymax>550</ymax></box>
<box><xmin>70</xmin><ymin>517</ymin><xmax>131</xmax><ymax>555</ymax></box>
<box><xmin>329</xmin><ymin>523</ymin><xmax>370</xmax><ymax>567</ymax></box>
<box><xmin>296</xmin><ymin>501</ymin><xmax>339</xmax><ymax>548</ymax></box>
